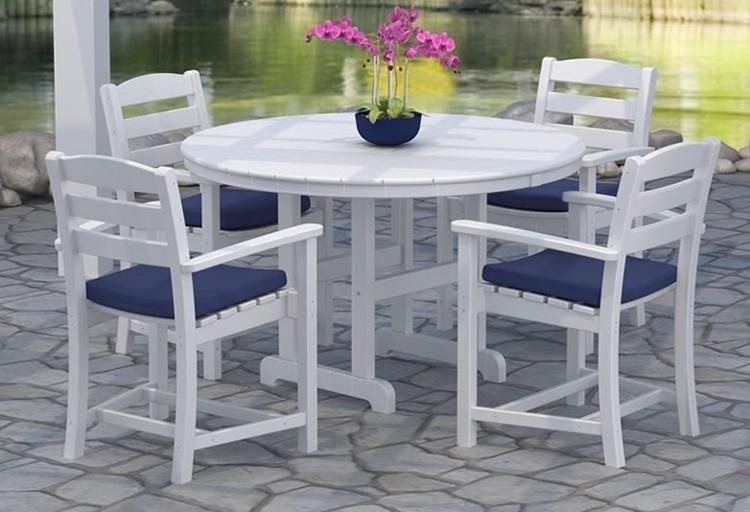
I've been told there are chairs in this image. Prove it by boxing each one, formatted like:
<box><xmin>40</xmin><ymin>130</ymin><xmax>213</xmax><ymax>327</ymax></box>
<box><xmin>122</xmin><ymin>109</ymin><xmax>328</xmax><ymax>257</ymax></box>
<box><xmin>451</xmin><ymin>138</ymin><xmax>722</xmax><ymax>467</ymax></box>
<box><xmin>438</xmin><ymin>57</ymin><xmax>657</xmax><ymax>356</ymax></box>
<box><xmin>99</xmin><ymin>70</ymin><xmax>331</xmax><ymax>380</ymax></box>
<box><xmin>44</xmin><ymin>150</ymin><xmax>323</xmax><ymax>485</ymax></box>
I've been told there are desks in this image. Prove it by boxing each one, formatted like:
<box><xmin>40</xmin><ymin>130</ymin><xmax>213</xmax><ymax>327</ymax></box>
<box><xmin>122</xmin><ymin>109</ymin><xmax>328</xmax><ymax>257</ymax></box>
<box><xmin>182</xmin><ymin>113</ymin><xmax>587</xmax><ymax>413</ymax></box>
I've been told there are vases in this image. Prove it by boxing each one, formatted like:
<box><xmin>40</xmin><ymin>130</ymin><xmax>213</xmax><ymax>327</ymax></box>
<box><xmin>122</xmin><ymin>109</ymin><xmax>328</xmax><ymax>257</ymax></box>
<box><xmin>355</xmin><ymin>110</ymin><xmax>422</xmax><ymax>146</ymax></box>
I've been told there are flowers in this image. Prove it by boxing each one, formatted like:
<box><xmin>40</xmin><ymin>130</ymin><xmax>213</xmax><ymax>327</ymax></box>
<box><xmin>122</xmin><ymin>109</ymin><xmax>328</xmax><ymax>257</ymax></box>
<box><xmin>305</xmin><ymin>5</ymin><xmax>461</xmax><ymax>123</ymax></box>
